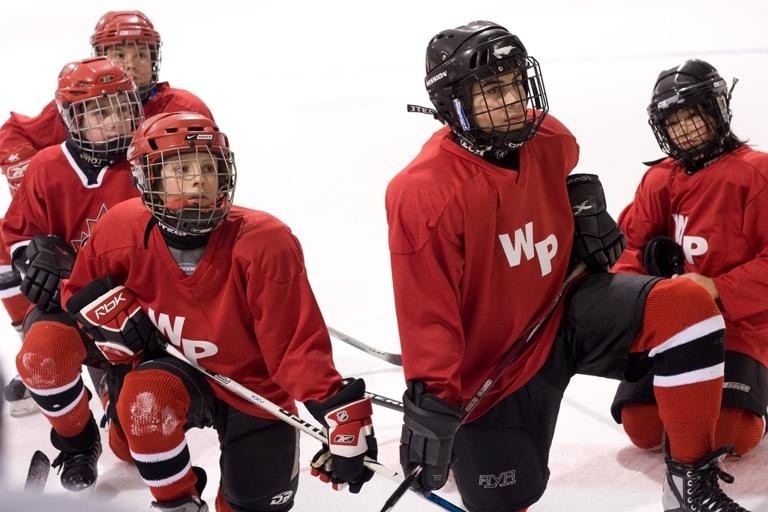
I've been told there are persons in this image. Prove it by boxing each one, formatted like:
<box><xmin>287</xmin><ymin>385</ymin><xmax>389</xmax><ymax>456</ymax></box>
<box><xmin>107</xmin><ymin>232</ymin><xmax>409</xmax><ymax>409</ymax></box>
<box><xmin>61</xmin><ymin>112</ymin><xmax>379</xmax><ymax>512</ymax></box>
<box><xmin>607</xmin><ymin>60</ymin><xmax>767</xmax><ymax>459</ymax></box>
<box><xmin>384</xmin><ymin>21</ymin><xmax>755</xmax><ymax>512</ymax></box>
<box><xmin>1</xmin><ymin>10</ymin><xmax>215</xmax><ymax>417</ymax></box>
<box><xmin>1</xmin><ymin>58</ymin><xmax>146</xmax><ymax>491</ymax></box>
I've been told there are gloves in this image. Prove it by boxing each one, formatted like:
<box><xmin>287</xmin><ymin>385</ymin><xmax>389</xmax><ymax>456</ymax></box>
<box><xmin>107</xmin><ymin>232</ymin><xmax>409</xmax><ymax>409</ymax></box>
<box><xmin>565</xmin><ymin>174</ymin><xmax>625</xmax><ymax>272</ymax></box>
<box><xmin>399</xmin><ymin>381</ymin><xmax>461</xmax><ymax>497</ymax></box>
<box><xmin>21</xmin><ymin>239</ymin><xmax>74</xmax><ymax>314</ymax></box>
<box><xmin>304</xmin><ymin>378</ymin><xmax>378</xmax><ymax>493</ymax></box>
<box><xmin>643</xmin><ymin>237</ymin><xmax>685</xmax><ymax>278</ymax></box>
<box><xmin>66</xmin><ymin>274</ymin><xmax>155</xmax><ymax>368</ymax></box>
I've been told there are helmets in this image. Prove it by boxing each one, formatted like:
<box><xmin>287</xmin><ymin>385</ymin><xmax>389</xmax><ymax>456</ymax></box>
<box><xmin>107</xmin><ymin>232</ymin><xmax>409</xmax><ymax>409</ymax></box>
<box><xmin>126</xmin><ymin>111</ymin><xmax>236</xmax><ymax>236</ymax></box>
<box><xmin>90</xmin><ymin>12</ymin><xmax>163</xmax><ymax>98</ymax></box>
<box><xmin>647</xmin><ymin>63</ymin><xmax>736</xmax><ymax>164</ymax></box>
<box><xmin>425</xmin><ymin>20</ymin><xmax>549</xmax><ymax>147</ymax></box>
<box><xmin>55</xmin><ymin>59</ymin><xmax>145</xmax><ymax>162</ymax></box>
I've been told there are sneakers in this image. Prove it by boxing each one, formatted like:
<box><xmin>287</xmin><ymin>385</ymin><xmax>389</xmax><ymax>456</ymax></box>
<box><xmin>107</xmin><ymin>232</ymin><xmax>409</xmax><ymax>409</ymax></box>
<box><xmin>152</xmin><ymin>467</ymin><xmax>208</xmax><ymax>512</ymax></box>
<box><xmin>51</xmin><ymin>418</ymin><xmax>101</xmax><ymax>491</ymax></box>
<box><xmin>661</xmin><ymin>432</ymin><xmax>752</xmax><ymax>511</ymax></box>
<box><xmin>5</xmin><ymin>376</ymin><xmax>29</xmax><ymax>401</ymax></box>
